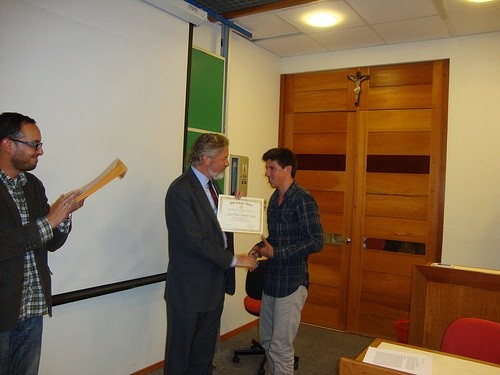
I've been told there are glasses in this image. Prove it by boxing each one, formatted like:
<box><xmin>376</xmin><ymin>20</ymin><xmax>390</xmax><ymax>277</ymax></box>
<box><xmin>3</xmin><ymin>136</ymin><xmax>43</xmax><ymax>150</ymax></box>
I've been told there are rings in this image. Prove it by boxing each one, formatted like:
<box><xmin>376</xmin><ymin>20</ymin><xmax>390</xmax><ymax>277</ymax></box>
<box><xmin>63</xmin><ymin>203</ymin><xmax>67</xmax><ymax>207</ymax></box>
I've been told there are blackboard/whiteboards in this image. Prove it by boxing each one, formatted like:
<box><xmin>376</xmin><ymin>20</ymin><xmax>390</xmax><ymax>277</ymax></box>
<box><xmin>182</xmin><ymin>45</ymin><xmax>226</xmax><ymax>195</ymax></box>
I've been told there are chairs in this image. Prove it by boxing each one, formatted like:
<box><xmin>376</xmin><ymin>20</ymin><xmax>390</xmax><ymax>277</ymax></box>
<box><xmin>393</xmin><ymin>319</ymin><xmax>409</xmax><ymax>344</ymax></box>
<box><xmin>440</xmin><ymin>318</ymin><xmax>500</xmax><ymax>365</ymax></box>
<box><xmin>233</xmin><ymin>296</ymin><xmax>300</xmax><ymax>375</ymax></box>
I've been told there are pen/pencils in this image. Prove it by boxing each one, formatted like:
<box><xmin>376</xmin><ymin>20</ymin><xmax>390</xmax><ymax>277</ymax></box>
<box><xmin>438</xmin><ymin>264</ymin><xmax>451</xmax><ymax>266</ymax></box>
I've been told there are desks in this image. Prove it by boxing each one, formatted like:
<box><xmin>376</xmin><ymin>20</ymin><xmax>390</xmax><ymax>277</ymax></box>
<box><xmin>409</xmin><ymin>261</ymin><xmax>500</xmax><ymax>351</ymax></box>
<box><xmin>339</xmin><ymin>337</ymin><xmax>500</xmax><ymax>375</ymax></box>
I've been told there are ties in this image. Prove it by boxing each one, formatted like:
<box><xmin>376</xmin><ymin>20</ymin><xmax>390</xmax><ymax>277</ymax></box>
<box><xmin>207</xmin><ymin>180</ymin><xmax>233</xmax><ymax>252</ymax></box>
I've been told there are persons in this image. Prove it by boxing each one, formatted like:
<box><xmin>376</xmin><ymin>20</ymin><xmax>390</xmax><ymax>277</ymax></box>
<box><xmin>0</xmin><ymin>112</ymin><xmax>86</xmax><ymax>374</ymax></box>
<box><xmin>163</xmin><ymin>133</ymin><xmax>258</xmax><ymax>375</ymax></box>
<box><xmin>248</xmin><ymin>147</ymin><xmax>325</xmax><ymax>375</ymax></box>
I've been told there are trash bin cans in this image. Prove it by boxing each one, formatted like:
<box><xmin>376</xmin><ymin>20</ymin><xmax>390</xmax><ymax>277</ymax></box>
<box><xmin>394</xmin><ymin>319</ymin><xmax>410</xmax><ymax>344</ymax></box>
